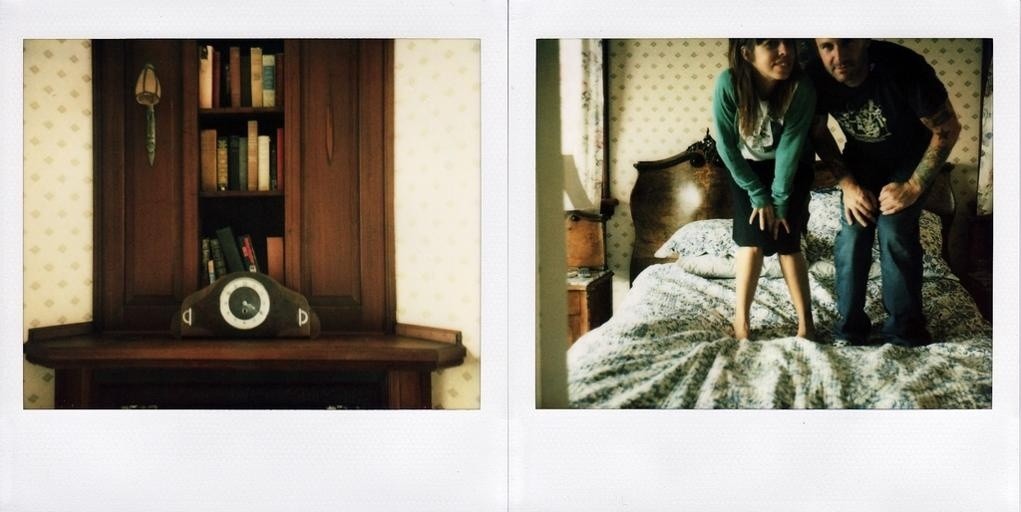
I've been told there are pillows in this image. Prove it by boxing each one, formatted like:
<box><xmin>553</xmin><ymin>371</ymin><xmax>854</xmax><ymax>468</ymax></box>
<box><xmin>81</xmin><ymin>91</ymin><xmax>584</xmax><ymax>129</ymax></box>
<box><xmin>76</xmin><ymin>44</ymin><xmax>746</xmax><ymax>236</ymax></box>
<box><xmin>655</xmin><ymin>182</ymin><xmax>945</xmax><ymax>279</ymax></box>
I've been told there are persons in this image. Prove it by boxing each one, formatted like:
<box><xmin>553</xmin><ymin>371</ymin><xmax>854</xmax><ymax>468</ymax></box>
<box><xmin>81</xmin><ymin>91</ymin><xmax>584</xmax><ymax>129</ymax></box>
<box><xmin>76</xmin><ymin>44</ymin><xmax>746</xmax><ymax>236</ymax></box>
<box><xmin>805</xmin><ymin>38</ymin><xmax>962</xmax><ymax>350</ymax></box>
<box><xmin>711</xmin><ymin>38</ymin><xmax>818</xmax><ymax>343</ymax></box>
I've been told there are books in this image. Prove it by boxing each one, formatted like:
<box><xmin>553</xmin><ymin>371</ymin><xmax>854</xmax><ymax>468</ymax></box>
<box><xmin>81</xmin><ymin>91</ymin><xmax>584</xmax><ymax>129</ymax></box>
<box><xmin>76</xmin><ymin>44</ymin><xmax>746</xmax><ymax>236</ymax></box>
<box><xmin>197</xmin><ymin>44</ymin><xmax>285</xmax><ymax>290</ymax></box>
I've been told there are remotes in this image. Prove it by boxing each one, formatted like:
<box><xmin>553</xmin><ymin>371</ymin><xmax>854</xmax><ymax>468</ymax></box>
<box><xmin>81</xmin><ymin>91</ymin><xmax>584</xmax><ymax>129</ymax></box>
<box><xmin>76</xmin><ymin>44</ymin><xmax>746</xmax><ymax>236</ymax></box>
<box><xmin>577</xmin><ymin>268</ymin><xmax>591</xmax><ymax>278</ymax></box>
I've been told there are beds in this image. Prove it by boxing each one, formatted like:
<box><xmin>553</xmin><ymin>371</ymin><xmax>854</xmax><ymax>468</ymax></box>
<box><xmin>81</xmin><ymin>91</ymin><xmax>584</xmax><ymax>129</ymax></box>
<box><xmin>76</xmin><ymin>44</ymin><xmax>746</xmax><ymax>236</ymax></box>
<box><xmin>564</xmin><ymin>127</ymin><xmax>993</xmax><ymax>410</ymax></box>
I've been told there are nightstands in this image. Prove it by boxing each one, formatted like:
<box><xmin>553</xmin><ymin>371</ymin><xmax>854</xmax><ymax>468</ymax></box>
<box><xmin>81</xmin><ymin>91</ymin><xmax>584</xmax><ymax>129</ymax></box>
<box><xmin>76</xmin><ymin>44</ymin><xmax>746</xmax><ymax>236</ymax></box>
<box><xmin>566</xmin><ymin>269</ymin><xmax>616</xmax><ymax>350</ymax></box>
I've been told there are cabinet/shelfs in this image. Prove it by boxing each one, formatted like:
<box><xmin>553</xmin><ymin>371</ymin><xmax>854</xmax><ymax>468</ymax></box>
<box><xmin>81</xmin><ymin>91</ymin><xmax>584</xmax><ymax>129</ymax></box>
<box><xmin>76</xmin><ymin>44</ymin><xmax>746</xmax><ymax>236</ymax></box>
<box><xmin>24</xmin><ymin>39</ymin><xmax>467</xmax><ymax>409</ymax></box>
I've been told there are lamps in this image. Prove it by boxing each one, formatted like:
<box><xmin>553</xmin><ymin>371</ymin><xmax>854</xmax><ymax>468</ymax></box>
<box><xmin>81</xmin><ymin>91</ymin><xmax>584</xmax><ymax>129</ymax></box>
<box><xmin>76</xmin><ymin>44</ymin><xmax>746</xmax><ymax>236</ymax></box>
<box><xmin>562</xmin><ymin>154</ymin><xmax>596</xmax><ymax>279</ymax></box>
<box><xmin>135</xmin><ymin>62</ymin><xmax>163</xmax><ymax>168</ymax></box>
